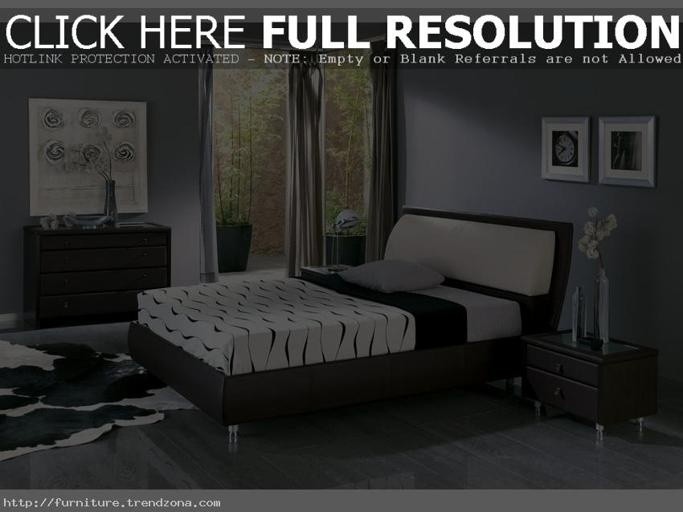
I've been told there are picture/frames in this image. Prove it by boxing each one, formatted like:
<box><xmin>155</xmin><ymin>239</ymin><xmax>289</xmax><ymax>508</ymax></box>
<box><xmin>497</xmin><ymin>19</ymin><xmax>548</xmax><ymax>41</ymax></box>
<box><xmin>541</xmin><ymin>116</ymin><xmax>590</xmax><ymax>184</ymax></box>
<box><xmin>598</xmin><ymin>115</ymin><xmax>656</xmax><ymax>189</ymax></box>
<box><xmin>26</xmin><ymin>96</ymin><xmax>150</xmax><ymax>219</ymax></box>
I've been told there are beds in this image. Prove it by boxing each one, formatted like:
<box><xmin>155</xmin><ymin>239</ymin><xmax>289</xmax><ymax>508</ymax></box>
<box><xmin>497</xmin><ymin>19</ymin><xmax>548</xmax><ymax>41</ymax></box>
<box><xmin>127</xmin><ymin>207</ymin><xmax>574</xmax><ymax>444</ymax></box>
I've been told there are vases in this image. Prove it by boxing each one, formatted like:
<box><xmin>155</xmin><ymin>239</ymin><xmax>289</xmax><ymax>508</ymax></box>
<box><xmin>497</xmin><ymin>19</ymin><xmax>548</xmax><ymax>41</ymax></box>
<box><xmin>572</xmin><ymin>285</ymin><xmax>587</xmax><ymax>343</ymax></box>
<box><xmin>594</xmin><ymin>270</ymin><xmax>610</xmax><ymax>344</ymax></box>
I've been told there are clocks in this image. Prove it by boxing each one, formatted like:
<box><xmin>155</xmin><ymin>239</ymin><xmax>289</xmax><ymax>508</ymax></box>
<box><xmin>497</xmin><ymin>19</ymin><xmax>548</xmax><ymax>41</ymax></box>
<box><xmin>553</xmin><ymin>133</ymin><xmax>578</xmax><ymax>166</ymax></box>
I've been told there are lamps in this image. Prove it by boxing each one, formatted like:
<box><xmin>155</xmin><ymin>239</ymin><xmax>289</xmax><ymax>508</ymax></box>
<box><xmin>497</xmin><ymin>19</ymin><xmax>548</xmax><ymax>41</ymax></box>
<box><xmin>328</xmin><ymin>209</ymin><xmax>362</xmax><ymax>271</ymax></box>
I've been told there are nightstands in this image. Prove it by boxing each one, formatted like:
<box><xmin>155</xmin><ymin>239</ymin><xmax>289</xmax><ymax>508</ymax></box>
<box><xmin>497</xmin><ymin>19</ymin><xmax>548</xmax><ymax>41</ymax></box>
<box><xmin>521</xmin><ymin>327</ymin><xmax>659</xmax><ymax>441</ymax></box>
<box><xmin>300</xmin><ymin>263</ymin><xmax>356</xmax><ymax>282</ymax></box>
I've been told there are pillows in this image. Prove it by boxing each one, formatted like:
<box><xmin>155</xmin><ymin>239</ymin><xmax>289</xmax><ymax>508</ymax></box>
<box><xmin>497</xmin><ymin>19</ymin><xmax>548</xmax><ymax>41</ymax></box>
<box><xmin>337</xmin><ymin>258</ymin><xmax>445</xmax><ymax>295</ymax></box>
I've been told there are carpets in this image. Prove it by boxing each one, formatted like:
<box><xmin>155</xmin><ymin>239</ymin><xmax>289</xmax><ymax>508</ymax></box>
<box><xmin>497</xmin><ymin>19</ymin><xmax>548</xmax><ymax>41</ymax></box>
<box><xmin>0</xmin><ymin>338</ymin><xmax>200</xmax><ymax>462</ymax></box>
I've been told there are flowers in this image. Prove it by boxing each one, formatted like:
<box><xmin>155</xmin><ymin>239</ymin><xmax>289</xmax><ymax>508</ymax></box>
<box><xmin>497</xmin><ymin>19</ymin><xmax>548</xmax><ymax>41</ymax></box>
<box><xmin>577</xmin><ymin>205</ymin><xmax>618</xmax><ymax>270</ymax></box>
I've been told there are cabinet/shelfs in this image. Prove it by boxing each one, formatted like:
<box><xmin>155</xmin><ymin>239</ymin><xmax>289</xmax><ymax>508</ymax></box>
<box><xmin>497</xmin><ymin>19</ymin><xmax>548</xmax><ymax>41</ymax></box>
<box><xmin>23</xmin><ymin>221</ymin><xmax>171</xmax><ymax>330</ymax></box>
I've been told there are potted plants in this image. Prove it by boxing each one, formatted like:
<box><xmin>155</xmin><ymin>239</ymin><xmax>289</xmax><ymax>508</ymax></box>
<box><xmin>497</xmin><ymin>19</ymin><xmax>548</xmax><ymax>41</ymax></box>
<box><xmin>92</xmin><ymin>144</ymin><xmax>118</xmax><ymax>226</ymax></box>
<box><xmin>211</xmin><ymin>44</ymin><xmax>288</xmax><ymax>273</ymax></box>
<box><xmin>323</xmin><ymin>42</ymin><xmax>375</xmax><ymax>267</ymax></box>
